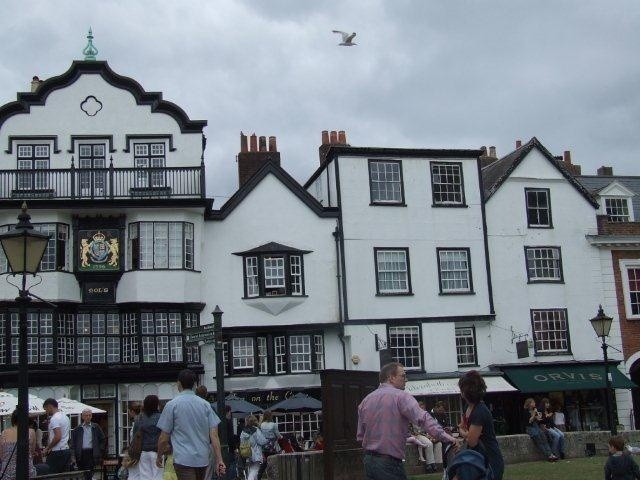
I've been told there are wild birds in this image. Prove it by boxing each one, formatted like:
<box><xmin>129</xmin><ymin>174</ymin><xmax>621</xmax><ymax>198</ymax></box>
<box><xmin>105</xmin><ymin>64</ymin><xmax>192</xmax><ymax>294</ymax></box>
<box><xmin>332</xmin><ymin>28</ymin><xmax>359</xmax><ymax>48</ymax></box>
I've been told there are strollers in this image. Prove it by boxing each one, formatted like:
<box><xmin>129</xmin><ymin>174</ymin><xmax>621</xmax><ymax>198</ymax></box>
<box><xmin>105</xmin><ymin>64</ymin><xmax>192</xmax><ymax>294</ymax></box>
<box><xmin>442</xmin><ymin>438</ymin><xmax>494</xmax><ymax>479</ymax></box>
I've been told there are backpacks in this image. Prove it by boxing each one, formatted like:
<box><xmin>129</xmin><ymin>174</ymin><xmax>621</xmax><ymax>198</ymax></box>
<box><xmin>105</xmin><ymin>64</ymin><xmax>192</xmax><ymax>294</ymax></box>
<box><xmin>238</xmin><ymin>437</ymin><xmax>253</xmax><ymax>459</ymax></box>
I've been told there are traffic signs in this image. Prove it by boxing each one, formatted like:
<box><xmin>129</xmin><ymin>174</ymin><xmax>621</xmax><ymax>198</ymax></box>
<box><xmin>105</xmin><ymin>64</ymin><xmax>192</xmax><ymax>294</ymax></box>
<box><xmin>183</xmin><ymin>324</ymin><xmax>215</xmax><ymax>342</ymax></box>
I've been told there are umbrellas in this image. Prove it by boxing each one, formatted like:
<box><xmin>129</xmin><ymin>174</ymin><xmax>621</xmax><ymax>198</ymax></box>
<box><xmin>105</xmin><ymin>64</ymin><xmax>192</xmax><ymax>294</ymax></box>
<box><xmin>0</xmin><ymin>391</ymin><xmax>47</xmax><ymax>418</ymax></box>
<box><xmin>263</xmin><ymin>392</ymin><xmax>322</xmax><ymax>438</ymax></box>
<box><xmin>210</xmin><ymin>394</ymin><xmax>263</xmax><ymax>417</ymax></box>
<box><xmin>53</xmin><ymin>397</ymin><xmax>107</xmax><ymax>414</ymax></box>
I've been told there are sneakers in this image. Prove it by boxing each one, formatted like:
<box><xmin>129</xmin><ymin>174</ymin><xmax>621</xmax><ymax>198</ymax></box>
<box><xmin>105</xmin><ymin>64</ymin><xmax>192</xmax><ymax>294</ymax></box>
<box><xmin>418</xmin><ymin>456</ymin><xmax>427</xmax><ymax>462</ymax></box>
<box><xmin>424</xmin><ymin>463</ymin><xmax>445</xmax><ymax>475</ymax></box>
<box><xmin>548</xmin><ymin>452</ymin><xmax>565</xmax><ymax>462</ymax></box>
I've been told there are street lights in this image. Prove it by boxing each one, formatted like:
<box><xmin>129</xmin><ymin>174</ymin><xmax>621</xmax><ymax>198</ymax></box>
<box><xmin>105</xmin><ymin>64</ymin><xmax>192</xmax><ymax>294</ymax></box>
<box><xmin>589</xmin><ymin>305</ymin><xmax>618</xmax><ymax>437</ymax></box>
<box><xmin>1</xmin><ymin>198</ymin><xmax>54</xmax><ymax>480</ymax></box>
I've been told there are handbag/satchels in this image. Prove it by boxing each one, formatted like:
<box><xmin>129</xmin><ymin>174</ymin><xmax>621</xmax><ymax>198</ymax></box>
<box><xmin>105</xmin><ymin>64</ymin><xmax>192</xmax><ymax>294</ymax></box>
<box><xmin>127</xmin><ymin>429</ymin><xmax>144</xmax><ymax>464</ymax></box>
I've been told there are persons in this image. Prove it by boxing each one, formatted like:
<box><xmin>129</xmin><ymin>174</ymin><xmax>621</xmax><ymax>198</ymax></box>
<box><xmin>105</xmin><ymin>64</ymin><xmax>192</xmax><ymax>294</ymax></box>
<box><xmin>196</xmin><ymin>384</ymin><xmax>208</xmax><ymax>400</ymax></box>
<box><xmin>239</xmin><ymin>415</ymin><xmax>269</xmax><ymax>480</ymax></box>
<box><xmin>0</xmin><ymin>407</ymin><xmax>39</xmax><ymax>479</ymax></box>
<box><xmin>355</xmin><ymin>362</ymin><xmax>461</xmax><ymax>480</ymax></box>
<box><xmin>155</xmin><ymin>368</ymin><xmax>227</xmax><ymax>480</ymax></box>
<box><xmin>522</xmin><ymin>397</ymin><xmax>558</xmax><ymax>463</ymax></box>
<box><xmin>405</xmin><ymin>400</ymin><xmax>457</xmax><ymax>474</ymax></box>
<box><xmin>41</xmin><ymin>397</ymin><xmax>72</xmax><ymax>474</ymax></box>
<box><xmin>260</xmin><ymin>411</ymin><xmax>283</xmax><ymax>452</ymax></box>
<box><xmin>133</xmin><ymin>394</ymin><xmax>165</xmax><ymax>480</ymax></box>
<box><xmin>456</xmin><ymin>369</ymin><xmax>506</xmax><ymax>480</ymax></box>
<box><xmin>71</xmin><ymin>408</ymin><xmax>108</xmax><ymax>480</ymax></box>
<box><xmin>27</xmin><ymin>419</ymin><xmax>44</xmax><ymax>464</ymax></box>
<box><xmin>604</xmin><ymin>435</ymin><xmax>640</xmax><ymax>480</ymax></box>
<box><xmin>114</xmin><ymin>445</ymin><xmax>128</xmax><ymax>480</ymax></box>
<box><xmin>538</xmin><ymin>397</ymin><xmax>565</xmax><ymax>461</ymax></box>
<box><xmin>226</xmin><ymin>405</ymin><xmax>237</xmax><ymax>455</ymax></box>
<box><xmin>127</xmin><ymin>402</ymin><xmax>144</xmax><ymax>446</ymax></box>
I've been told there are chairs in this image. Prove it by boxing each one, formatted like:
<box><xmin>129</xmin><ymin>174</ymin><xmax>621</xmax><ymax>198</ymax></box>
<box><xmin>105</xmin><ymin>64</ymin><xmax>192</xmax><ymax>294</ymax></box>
<box><xmin>100</xmin><ymin>452</ymin><xmax>123</xmax><ymax>480</ymax></box>
